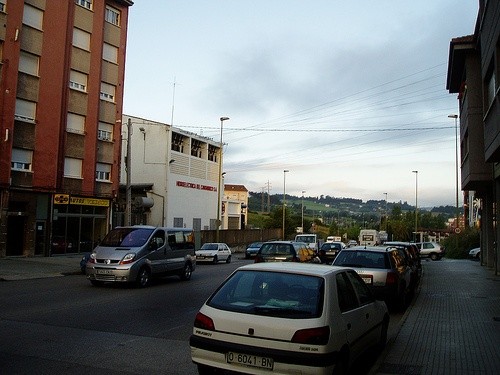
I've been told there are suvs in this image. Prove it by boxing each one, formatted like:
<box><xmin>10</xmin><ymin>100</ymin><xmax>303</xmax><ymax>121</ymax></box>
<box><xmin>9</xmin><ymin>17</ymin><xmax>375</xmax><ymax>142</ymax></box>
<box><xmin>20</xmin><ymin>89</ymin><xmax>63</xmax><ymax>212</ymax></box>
<box><xmin>414</xmin><ymin>242</ymin><xmax>445</xmax><ymax>261</ymax></box>
<box><xmin>382</xmin><ymin>242</ymin><xmax>420</xmax><ymax>276</ymax></box>
<box><xmin>331</xmin><ymin>246</ymin><xmax>414</xmax><ymax>303</ymax></box>
<box><xmin>320</xmin><ymin>242</ymin><xmax>347</xmax><ymax>264</ymax></box>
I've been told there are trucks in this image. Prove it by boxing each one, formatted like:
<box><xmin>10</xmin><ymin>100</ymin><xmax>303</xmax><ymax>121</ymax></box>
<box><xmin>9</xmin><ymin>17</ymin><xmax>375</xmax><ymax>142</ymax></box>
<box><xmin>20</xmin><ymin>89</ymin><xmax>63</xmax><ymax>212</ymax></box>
<box><xmin>294</xmin><ymin>234</ymin><xmax>324</xmax><ymax>254</ymax></box>
<box><xmin>326</xmin><ymin>236</ymin><xmax>341</xmax><ymax>242</ymax></box>
<box><xmin>358</xmin><ymin>230</ymin><xmax>382</xmax><ymax>247</ymax></box>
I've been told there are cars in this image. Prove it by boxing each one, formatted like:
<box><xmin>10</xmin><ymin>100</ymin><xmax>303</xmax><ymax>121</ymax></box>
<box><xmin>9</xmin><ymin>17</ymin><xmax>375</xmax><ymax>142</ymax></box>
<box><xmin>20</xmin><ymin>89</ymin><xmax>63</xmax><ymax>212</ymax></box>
<box><xmin>253</xmin><ymin>241</ymin><xmax>322</xmax><ymax>264</ymax></box>
<box><xmin>244</xmin><ymin>241</ymin><xmax>265</xmax><ymax>259</ymax></box>
<box><xmin>196</xmin><ymin>242</ymin><xmax>232</xmax><ymax>264</ymax></box>
<box><xmin>469</xmin><ymin>248</ymin><xmax>480</xmax><ymax>260</ymax></box>
<box><xmin>348</xmin><ymin>240</ymin><xmax>357</xmax><ymax>248</ymax></box>
<box><xmin>189</xmin><ymin>259</ymin><xmax>389</xmax><ymax>375</ymax></box>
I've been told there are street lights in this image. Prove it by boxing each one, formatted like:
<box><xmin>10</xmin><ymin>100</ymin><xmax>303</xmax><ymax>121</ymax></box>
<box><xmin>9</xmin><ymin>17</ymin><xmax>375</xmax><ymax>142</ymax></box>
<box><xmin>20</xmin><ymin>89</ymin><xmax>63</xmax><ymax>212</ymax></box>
<box><xmin>301</xmin><ymin>191</ymin><xmax>306</xmax><ymax>234</ymax></box>
<box><xmin>383</xmin><ymin>192</ymin><xmax>387</xmax><ymax>233</ymax></box>
<box><xmin>412</xmin><ymin>170</ymin><xmax>418</xmax><ymax>244</ymax></box>
<box><xmin>282</xmin><ymin>170</ymin><xmax>289</xmax><ymax>240</ymax></box>
<box><xmin>216</xmin><ymin>117</ymin><xmax>230</xmax><ymax>243</ymax></box>
<box><xmin>447</xmin><ymin>114</ymin><xmax>459</xmax><ymax>230</ymax></box>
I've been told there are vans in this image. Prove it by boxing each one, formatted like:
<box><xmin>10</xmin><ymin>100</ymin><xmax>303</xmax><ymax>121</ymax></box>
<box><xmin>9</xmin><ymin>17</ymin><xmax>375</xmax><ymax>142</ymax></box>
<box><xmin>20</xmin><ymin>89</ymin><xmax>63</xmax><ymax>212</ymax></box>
<box><xmin>86</xmin><ymin>224</ymin><xmax>195</xmax><ymax>287</ymax></box>
